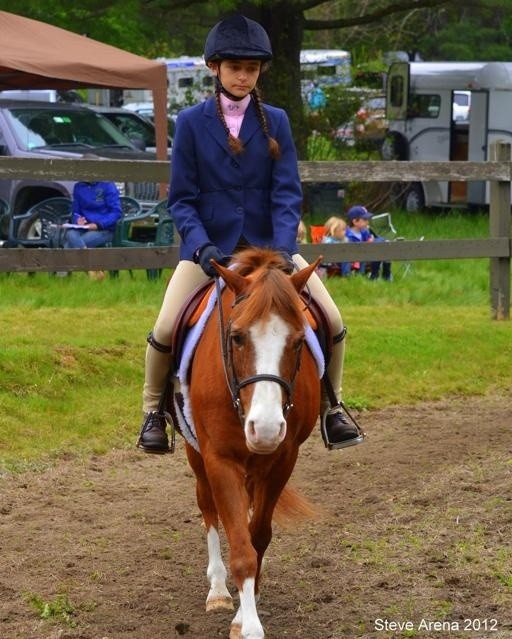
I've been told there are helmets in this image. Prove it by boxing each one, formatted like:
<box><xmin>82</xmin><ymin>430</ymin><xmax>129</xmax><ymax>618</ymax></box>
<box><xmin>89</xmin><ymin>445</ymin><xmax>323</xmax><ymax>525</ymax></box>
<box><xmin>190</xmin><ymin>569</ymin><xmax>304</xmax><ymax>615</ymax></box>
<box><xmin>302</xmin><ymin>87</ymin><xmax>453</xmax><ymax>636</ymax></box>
<box><xmin>204</xmin><ymin>14</ymin><xmax>274</xmax><ymax>62</ymax></box>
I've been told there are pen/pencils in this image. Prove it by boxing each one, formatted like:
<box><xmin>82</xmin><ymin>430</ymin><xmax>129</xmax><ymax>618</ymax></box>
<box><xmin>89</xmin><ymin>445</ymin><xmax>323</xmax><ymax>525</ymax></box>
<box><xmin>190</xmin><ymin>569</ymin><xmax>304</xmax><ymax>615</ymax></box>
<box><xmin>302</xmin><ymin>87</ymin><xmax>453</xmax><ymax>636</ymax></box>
<box><xmin>74</xmin><ymin>213</ymin><xmax>88</xmax><ymax>224</ymax></box>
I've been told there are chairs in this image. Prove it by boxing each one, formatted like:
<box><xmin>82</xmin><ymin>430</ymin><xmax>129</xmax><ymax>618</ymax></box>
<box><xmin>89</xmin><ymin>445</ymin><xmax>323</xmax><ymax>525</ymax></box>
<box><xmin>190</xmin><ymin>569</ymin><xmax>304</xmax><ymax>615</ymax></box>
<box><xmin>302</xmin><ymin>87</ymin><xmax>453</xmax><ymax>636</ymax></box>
<box><xmin>0</xmin><ymin>195</ymin><xmax>174</xmax><ymax>249</ymax></box>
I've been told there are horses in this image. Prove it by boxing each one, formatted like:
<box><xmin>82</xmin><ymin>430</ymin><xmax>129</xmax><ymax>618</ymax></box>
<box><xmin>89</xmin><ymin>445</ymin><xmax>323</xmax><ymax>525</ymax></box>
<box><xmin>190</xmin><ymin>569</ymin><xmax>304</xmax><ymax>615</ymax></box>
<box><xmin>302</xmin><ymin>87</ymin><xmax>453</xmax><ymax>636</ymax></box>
<box><xmin>160</xmin><ymin>244</ymin><xmax>328</xmax><ymax>639</ymax></box>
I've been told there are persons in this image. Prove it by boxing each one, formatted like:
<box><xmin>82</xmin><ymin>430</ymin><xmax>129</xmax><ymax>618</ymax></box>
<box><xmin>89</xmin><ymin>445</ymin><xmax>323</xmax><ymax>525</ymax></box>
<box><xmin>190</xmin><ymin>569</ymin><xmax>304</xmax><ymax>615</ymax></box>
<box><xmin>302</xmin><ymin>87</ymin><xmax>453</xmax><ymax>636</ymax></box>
<box><xmin>305</xmin><ymin>79</ymin><xmax>327</xmax><ymax>112</ymax></box>
<box><xmin>294</xmin><ymin>218</ymin><xmax>308</xmax><ymax>244</ymax></box>
<box><xmin>140</xmin><ymin>14</ymin><xmax>359</xmax><ymax>454</ymax></box>
<box><xmin>318</xmin><ymin>215</ymin><xmax>367</xmax><ymax>279</ymax></box>
<box><xmin>60</xmin><ymin>151</ymin><xmax>125</xmax><ymax>283</ymax></box>
<box><xmin>343</xmin><ymin>205</ymin><xmax>393</xmax><ymax>283</ymax></box>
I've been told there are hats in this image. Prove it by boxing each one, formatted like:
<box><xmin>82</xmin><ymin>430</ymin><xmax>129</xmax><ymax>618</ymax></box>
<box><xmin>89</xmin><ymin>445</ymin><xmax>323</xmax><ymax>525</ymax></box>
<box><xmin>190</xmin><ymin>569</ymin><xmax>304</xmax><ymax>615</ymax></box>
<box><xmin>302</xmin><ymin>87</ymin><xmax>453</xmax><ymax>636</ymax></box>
<box><xmin>347</xmin><ymin>205</ymin><xmax>373</xmax><ymax>219</ymax></box>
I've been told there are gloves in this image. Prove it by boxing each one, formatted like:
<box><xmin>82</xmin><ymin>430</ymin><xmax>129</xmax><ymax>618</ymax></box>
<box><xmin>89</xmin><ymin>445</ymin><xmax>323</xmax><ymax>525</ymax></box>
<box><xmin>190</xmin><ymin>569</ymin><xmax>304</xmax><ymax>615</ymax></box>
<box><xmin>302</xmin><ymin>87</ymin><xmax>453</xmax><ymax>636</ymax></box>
<box><xmin>277</xmin><ymin>250</ymin><xmax>294</xmax><ymax>274</ymax></box>
<box><xmin>198</xmin><ymin>243</ymin><xmax>227</xmax><ymax>277</ymax></box>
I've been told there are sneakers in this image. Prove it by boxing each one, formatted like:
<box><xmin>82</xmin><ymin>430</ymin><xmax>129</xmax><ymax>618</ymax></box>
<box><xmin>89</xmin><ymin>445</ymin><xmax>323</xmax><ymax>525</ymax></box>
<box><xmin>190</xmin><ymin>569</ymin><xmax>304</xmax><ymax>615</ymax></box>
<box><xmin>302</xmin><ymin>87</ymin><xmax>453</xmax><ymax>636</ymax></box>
<box><xmin>320</xmin><ymin>413</ymin><xmax>358</xmax><ymax>446</ymax></box>
<box><xmin>142</xmin><ymin>415</ymin><xmax>169</xmax><ymax>450</ymax></box>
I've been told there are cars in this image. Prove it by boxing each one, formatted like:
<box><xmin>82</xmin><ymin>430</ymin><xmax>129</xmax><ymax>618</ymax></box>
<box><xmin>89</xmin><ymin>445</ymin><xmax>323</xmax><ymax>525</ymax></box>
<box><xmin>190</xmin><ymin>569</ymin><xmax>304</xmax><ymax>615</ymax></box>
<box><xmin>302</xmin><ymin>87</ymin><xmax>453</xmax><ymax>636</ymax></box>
<box><xmin>0</xmin><ymin>99</ymin><xmax>177</xmax><ymax>247</ymax></box>
<box><xmin>330</xmin><ymin>94</ymin><xmax>389</xmax><ymax>152</ymax></box>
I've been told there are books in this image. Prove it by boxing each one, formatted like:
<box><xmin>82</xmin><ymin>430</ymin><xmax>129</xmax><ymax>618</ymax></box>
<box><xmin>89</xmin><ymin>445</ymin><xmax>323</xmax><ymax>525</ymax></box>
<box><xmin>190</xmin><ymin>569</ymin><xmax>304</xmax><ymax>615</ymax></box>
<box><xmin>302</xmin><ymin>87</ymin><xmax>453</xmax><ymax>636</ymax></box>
<box><xmin>62</xmin><ymin>223</ymin><xmax>90</xmax><ymax>230</ymax></box>
